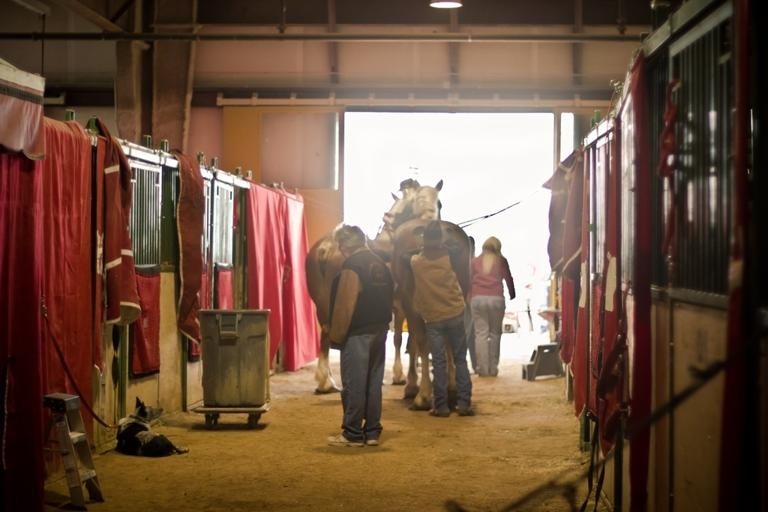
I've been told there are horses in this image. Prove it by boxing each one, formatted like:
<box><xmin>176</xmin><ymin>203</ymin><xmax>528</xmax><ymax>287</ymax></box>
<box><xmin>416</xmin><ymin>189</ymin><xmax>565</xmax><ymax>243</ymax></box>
<box><xmin>304</xmin><ymin>178</ymin><xmax>474</xmax><ymax>411</ymax></box>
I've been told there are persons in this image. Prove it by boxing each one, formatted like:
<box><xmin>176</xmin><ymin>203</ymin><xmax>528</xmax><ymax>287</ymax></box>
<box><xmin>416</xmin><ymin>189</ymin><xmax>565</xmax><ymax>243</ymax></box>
<box><xmin>470</xmin><ymin>236</ymin><xmax>515</xmax><ymax>378</ymax></box>
<box><xmin>328</xmin><ymin>225</ymin><xmax>394</xmax><ymax>447</ymax></box>
<box><xmin>399</xmin><ymin>221</ymin><xmax>475</xmax><ymax>417</ymax></box>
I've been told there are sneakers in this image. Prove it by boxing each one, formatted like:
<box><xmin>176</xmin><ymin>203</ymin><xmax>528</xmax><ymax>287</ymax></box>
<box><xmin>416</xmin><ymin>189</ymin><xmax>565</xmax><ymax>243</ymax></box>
<box><xmin>478</xmin><ymin>369</ymin><xmax>498</xmax><ymax>376</ymax></box>
<box><xmin>429</xmin><ymin>408</ymin><xmax>474</xmax><ymax>417</ymax></box>
<box><xmin>328</xmin><ymin>432</ymin><xmax>379</xmax><ymax>447</ymax></box>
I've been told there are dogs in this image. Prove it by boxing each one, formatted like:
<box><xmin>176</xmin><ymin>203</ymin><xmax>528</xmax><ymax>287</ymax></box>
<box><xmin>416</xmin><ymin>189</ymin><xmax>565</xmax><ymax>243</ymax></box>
<box><xmin>115</xmin><ymin>396</ymin><xmax>191</xmax><ymax>457</ymax></box>
